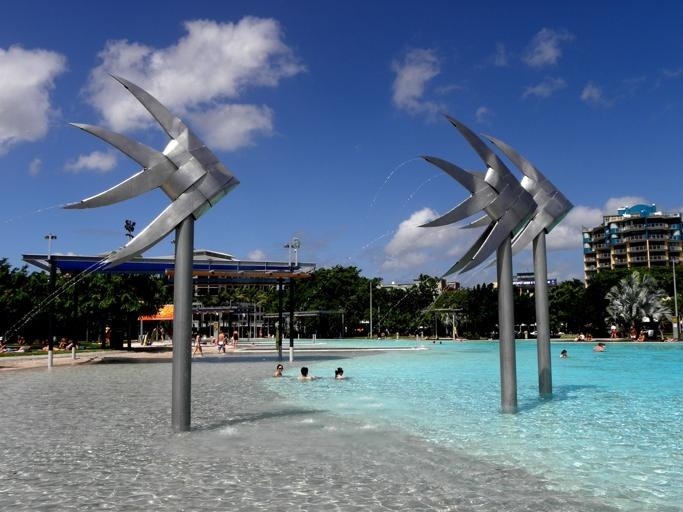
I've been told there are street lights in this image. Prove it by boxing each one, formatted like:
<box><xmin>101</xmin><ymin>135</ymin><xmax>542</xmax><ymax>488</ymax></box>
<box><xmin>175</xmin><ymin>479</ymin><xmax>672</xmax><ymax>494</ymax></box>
<box><xmin>44</xmin><ymin>234</ymin><xmax>56</xmax><ymax>259</ymax></box>
<box><xmin>124</xmin><ymin>217</ymin><xmax>134</xmax><ymax>241</ymax></box>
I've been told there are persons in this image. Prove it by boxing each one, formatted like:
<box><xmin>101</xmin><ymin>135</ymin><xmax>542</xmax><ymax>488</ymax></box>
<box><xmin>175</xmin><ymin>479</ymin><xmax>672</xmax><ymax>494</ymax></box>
<box><xmin>273</xmin><ymin>363</ymin><xmax>347</xmax><ymax>380</ymax></box>
<box><xmin>591</xmin><ymin>342</ymin><xmax>606</xmax><ymax>353</ymax></box>
<box><xmin>0</xmin><ymin>334</ymin><xmax>81</xmax><ymax>353</ymax></box>
<box><xmin>560</xmin><ymin>349</ymin><xmax>568</xmax><ymax>361</ymax></box>
<box><xmin>611</xmin><ymin>322</ymin><xmax>645</xmax><ymax>342</ymax></box>
<box><xmin>141</xmin><ymin>324</ymin><xmax>165</xmax><ymax>346</ymax></box>
<box><xmin>574</xmin><ymin>331</ymin><xmax>592</xmax><ymax>342</ymax></box>
<box><xmin>191</xmin><ymin>329</ymin><xmax>240</xmax><ymax>356</ymax></box>
